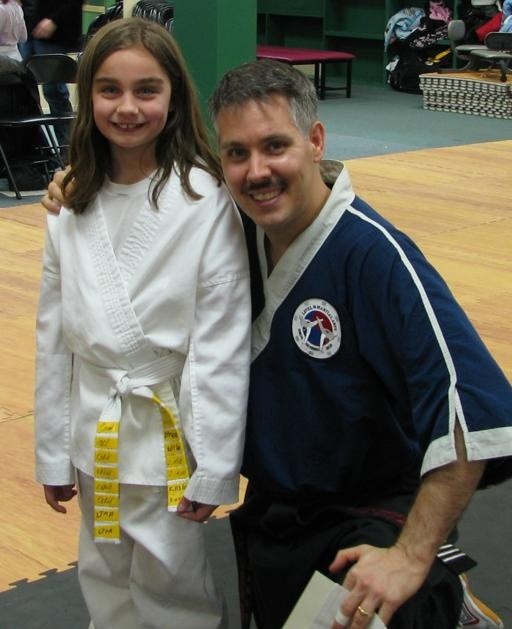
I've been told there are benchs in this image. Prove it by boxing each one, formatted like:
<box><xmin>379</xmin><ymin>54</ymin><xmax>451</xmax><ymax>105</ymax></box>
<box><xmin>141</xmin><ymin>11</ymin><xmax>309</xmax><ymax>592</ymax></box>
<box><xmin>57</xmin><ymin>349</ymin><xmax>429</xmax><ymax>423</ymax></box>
<box><xmin>257</xmin><ymin>45</ymin><xmax>355</xmax><ymax>100</ymax></box>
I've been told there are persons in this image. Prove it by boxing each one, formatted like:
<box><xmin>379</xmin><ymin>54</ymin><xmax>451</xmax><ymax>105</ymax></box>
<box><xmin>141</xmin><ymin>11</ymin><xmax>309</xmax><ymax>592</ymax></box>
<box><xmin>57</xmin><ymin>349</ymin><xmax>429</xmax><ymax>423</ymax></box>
<box><xmin>15</xmin><ymin>0</ymin><xmax>84</xmax><ymax>171</ymax></box>
<box><xmin>31</xmin><ymin>16</ymin><xmax>253</xmax><ymax>629</ymax></box>
<box><xmin>0</xmin><ymin>0</ymin><xmax>30</xmax><ymax>62</ymax></box>
<box><xmin>40</xmin><ymin>55</ymin><xmax>511</xmax><ymax>629</ymax></box>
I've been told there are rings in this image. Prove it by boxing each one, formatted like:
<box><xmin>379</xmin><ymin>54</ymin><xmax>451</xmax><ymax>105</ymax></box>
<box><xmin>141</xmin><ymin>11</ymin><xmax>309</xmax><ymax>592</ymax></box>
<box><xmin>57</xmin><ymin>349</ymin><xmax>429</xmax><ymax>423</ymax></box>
<box><xmin>357</xmin><ymin>606</ymin><xmax>374</xmax><ymax>621</ymax></box>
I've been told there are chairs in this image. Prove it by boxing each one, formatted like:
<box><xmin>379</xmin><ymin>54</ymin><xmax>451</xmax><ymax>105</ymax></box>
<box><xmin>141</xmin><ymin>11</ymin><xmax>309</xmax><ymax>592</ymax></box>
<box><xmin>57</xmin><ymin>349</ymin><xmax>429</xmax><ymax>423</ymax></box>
<box><xmin>0</xmin><ymin>51</ymin><xmax>85</xmax><ymax>199</ymax></box>
<box><xmin>430</xmin><ymin>20</ymin><xmax>512</xmax><ymax>82</ymax></box>
